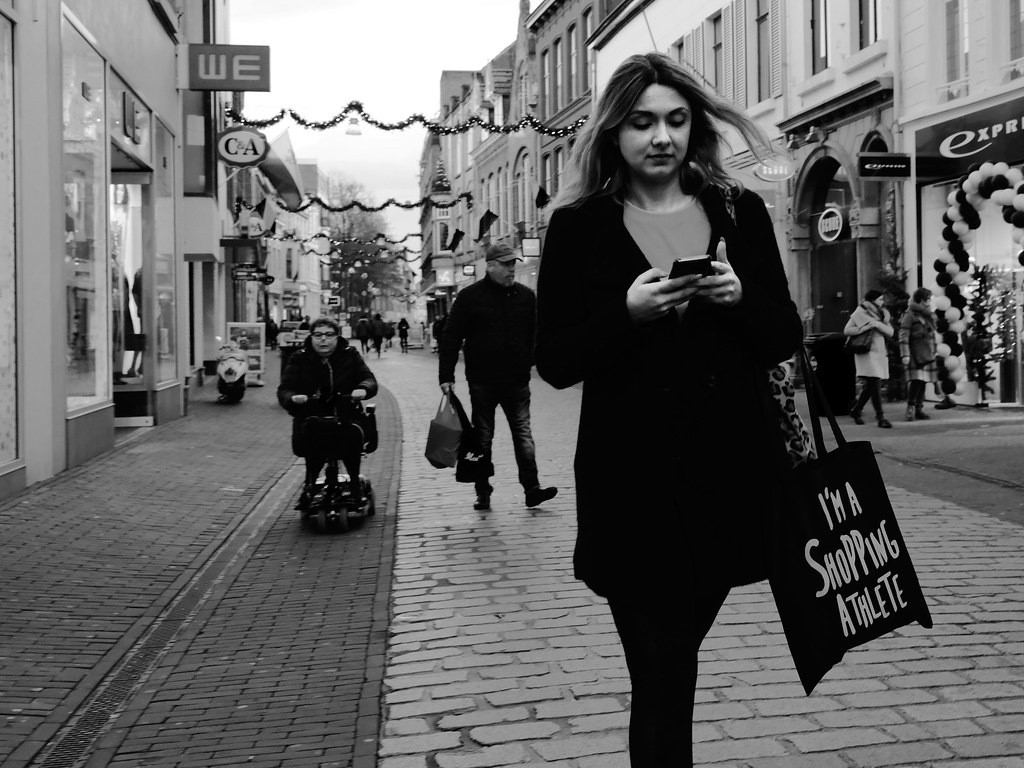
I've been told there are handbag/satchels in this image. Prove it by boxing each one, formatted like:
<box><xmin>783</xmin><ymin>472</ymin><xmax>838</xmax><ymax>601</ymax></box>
<box><xmin>746</xmin><ymin>310</ymin><xmax>934</xmax><ymax>696</ymax></box>
<box><xmin>844</xmin><ymin>305</ymin><xmax>875</xmax><ymax>354</ymax></box>
<box><xmin>726</xmin><ymin>183</ymin><xmax>816</xmax><ymax>465</ymax></box>
<box><xmin>424</xmin><ymin>392</ymin><xmax>462</xmax><ymax>469</ymax></box>
<box><xmin>448</xmin><ymin>384</ymin><xmax>494</xmax><ymax>483</ymax></box>
<box><xmin>359</xmin><ymin>407</ymin><xmax>378</xmax><ymax>454</ymax></box>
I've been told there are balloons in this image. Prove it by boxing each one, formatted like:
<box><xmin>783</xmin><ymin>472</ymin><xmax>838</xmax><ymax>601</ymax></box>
<box><xmin>933</xmin><ymin>161</ymin><xmax>1024</xmax><ymax>395</ymax></box>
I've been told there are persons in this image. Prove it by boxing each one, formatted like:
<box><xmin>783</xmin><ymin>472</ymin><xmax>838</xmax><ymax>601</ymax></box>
<box><xmin>397</xmin><ymin>318</ymin><xmax>410</xmax><ymax>353</ymax></box>
<box><xmin>299</xmin><ymin>315</ymin><xmax>311</xmax><ymax>330</ymax></box>
<box><xmin>534</xmin><ymin>53</ymin><xmax>805</xmax><ymax>768</ymax></box>
<box><xmin>356</xmin><ymin>313</ymin><xmax>395</xmax><ymax>357</ymax></box>
<box><xmin>899</xmin><ymin>287</ymin><xmax>938</xmax><ymax>421</ymax></box>
<box><xmin>278</xmin><ymin>317</ymin><xmax>378</xmax><ymax>508</ymax></box>
<box><xmin>439</xmin><ymin>244</ymin><xmax>559</xmax><ymax>509</ymax></box>
<box><xmin>844</xmin><ymin>290</ymin><xmax>894</xmax><ymax>428</ymax></box>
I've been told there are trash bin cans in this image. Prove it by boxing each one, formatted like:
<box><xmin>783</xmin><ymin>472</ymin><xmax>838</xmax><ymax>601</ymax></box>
<box><xmin>803</xmin><ymin>331</ymin><xmax>858</xmax><ymax>417</ymax></box>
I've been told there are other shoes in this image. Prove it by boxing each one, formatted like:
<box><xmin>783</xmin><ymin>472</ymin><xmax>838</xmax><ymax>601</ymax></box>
<box><xmin>878</xmin><ymin>420</ymin><xmax>892</xmax><ymax>428</ymax></box>
<box><xmin>525</xmin><ymin>487</ymin><xmax>558</xmax><ymax>507</ymax></box>
<box><xmin>915</xmin><ymin>412</ymin><xmax>930</xmax><ymax>419</ymax></box>
<box><xmin>473</xmin><ymin>493</ymin><xmax>490</xmax><ymax>510</ymax></box>
<box><xmin>850</xmin><ymin>408</ymin><xmax>865</xmax><ymax>424</ymax></box>
<box><xmin>350</xmin><ymin>476</ymin><xmax>362</xmax><ymax>501</ymax></box>
<box><xmin>904</xmin><ymin>404</ymin><xmax>915</xmax><ymax>420</ymax></box>
<box><xmin>300</xmin><ymin>480</ymin><xmax>316</xmax><ymax>502</ymax></box>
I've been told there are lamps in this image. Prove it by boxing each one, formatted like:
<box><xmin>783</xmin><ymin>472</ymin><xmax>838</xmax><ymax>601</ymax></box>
<box><xmin>787</xmin><ymin>133</ymin><xmax>805</xmax><ymax>148</ymax></box>
<box><xmin>806</xmin><ymin>126</ymin><xmax>837</xmax><ymax>142</ymax></box>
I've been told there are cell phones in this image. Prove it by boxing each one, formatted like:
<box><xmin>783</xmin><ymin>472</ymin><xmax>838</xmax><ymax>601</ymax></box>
<box><xmin>666</xmin><ymin>254</ymin><xmax>711</xmax><ymax>293</ymax></box>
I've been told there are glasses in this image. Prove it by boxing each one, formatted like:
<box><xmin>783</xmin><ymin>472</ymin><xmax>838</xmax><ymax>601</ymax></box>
<box><xmin>924</xmin><ymin>297</ymin><xmax>931</xmax><ymax>302</ymax></box>
<box><xmin>310</xmin><ymin>331</ymin><xmax>337</xmax><ymax>339</ymax></box>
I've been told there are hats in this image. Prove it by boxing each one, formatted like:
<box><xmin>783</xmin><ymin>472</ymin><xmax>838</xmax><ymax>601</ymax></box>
<box><xmin>486</xmin><ymin>241</ymin><xmax>524</xmax><ymax>263</ymax></box>
<box><xmin>865</xmin><ymin>290</ymin><xmax>883</xmax><ymax>303</ymax></box>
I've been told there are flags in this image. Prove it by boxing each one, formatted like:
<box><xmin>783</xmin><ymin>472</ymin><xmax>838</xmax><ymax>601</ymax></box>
<box><xmin>536</xmin><ymin>187</ymin><xmax>550</xmax><ymax>209</ymax></box>
<box><xmin>474</xmin><ymin>210</ymin><xmax>499</xmax><ymax>243</ymax></box>
<box><xmin>446</xmin><ymin>230</ymin><xmax>465</xmax><ymax>252</ymax></box>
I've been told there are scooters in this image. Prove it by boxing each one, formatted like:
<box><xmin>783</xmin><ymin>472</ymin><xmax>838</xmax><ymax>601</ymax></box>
<box><xmin>292</xmin><ymin>389</ymin><xmax>376</xmax><ymax>534</ymax></box>
